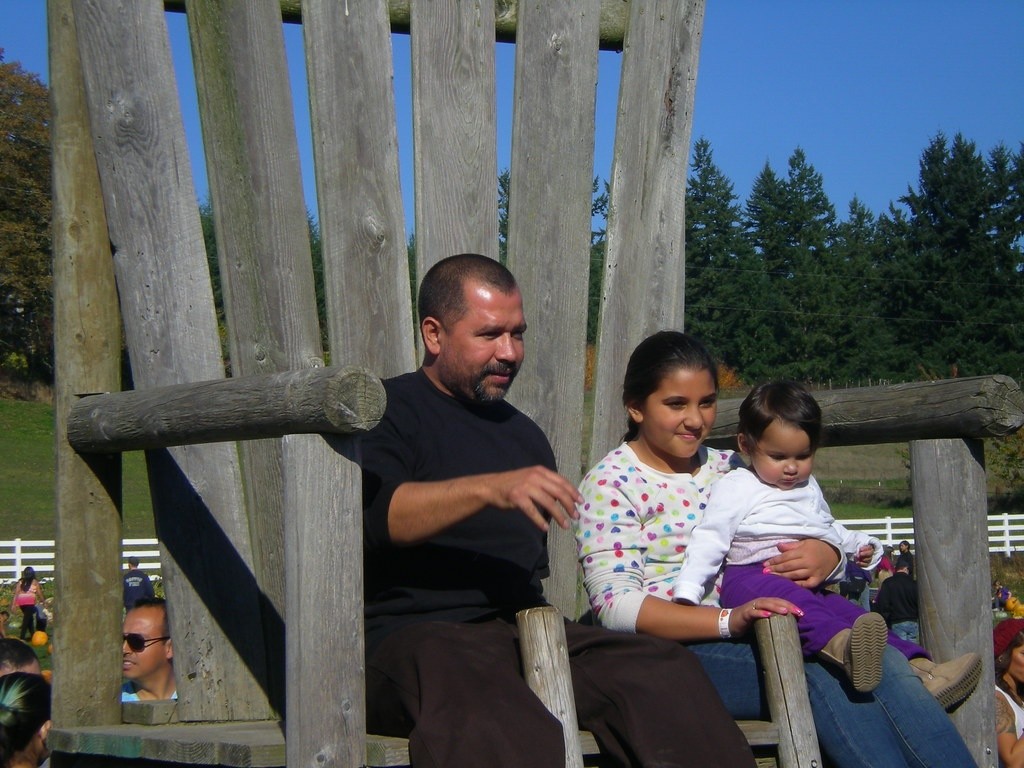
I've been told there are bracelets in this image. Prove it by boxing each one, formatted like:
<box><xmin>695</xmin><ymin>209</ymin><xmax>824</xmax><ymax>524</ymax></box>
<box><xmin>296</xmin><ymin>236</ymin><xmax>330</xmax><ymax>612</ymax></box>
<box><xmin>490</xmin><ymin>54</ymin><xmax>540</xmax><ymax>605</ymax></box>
<box><xmin>718</xmin><ymin>608</ymin><xmax>732</xmax><ymax>639</ymax></box>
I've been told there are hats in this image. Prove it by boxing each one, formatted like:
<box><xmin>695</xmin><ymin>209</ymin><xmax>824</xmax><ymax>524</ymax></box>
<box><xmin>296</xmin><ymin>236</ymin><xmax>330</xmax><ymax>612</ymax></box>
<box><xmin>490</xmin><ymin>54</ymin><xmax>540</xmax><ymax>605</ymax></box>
<box><xmin>896</xmin><ymin>560</ymin><xmax>909</xmax><ymax>566</ymax></box>
<box><xmin>993</xmin><ymin>617</ymin><xmax>1024</xmax><ymax>659</ymax></box>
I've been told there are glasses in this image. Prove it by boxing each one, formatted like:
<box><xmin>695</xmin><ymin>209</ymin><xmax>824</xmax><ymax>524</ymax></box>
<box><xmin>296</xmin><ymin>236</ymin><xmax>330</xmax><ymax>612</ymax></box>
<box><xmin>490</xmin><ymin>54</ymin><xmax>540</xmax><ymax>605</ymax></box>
<box><xmin>122</xmin><ymin>633</ymin><xmax>170</xmax><ymax>652</ymax></box>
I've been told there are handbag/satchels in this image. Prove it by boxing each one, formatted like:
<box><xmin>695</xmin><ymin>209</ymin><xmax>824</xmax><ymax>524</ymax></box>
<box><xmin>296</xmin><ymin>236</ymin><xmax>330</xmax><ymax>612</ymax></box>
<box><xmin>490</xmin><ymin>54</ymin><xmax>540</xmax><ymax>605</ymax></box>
<box><xmin>847</xmin><ymin>577</ymin><xmax>866</xmax><ymax>593</ymax></box>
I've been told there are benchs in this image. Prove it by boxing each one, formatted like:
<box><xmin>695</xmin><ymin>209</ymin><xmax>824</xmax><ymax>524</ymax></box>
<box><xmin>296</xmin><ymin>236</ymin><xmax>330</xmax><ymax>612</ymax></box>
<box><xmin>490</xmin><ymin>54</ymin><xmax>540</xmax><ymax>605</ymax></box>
<box><xmin>37</xmin><ymin>1</ymin><xmax>1023</xmax><ymax>768</ymax></box>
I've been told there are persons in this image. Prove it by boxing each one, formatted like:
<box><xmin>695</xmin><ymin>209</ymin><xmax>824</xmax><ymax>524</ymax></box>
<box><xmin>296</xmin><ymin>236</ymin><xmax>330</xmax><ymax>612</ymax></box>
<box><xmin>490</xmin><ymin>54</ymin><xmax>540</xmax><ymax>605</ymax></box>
<box><xmin>574</xmin><ymin>331</ymin><xmax>1024</xmax><ymax>768</ymax></box>
<box><xmin>360</xmin><ymin>255</ymin><xmax>759</xmax><ymax>768</ymax></box>
<box><xmin>0</xmin><ymin>558</ymin><xmax>178</xmax><ymax>767</ymax></box>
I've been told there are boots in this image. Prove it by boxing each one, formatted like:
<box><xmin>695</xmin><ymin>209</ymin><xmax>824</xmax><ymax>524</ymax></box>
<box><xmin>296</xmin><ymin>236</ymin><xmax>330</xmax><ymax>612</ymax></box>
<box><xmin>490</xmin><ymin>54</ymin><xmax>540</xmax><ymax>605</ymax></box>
<box><xmin>907</xmin><ymin>652</ymin><xmax>982</xmax><ymax>710</ymax></box>
<box><xmin>815</xmin><ymin>612</ymin><xmax>888</xmax><ymax>692</ymax></box>
